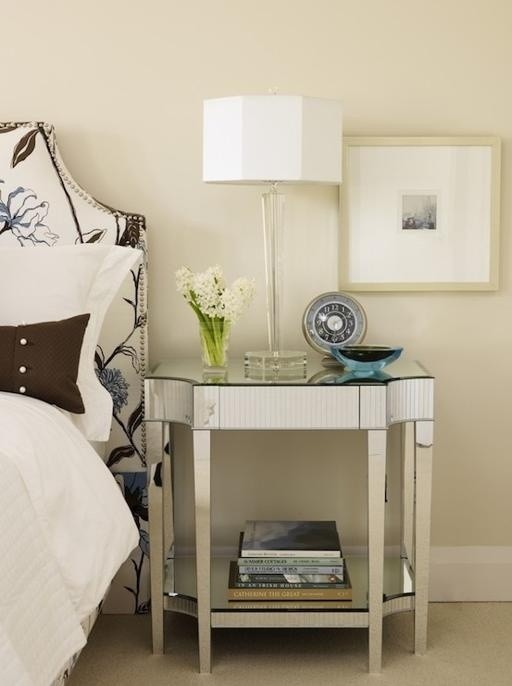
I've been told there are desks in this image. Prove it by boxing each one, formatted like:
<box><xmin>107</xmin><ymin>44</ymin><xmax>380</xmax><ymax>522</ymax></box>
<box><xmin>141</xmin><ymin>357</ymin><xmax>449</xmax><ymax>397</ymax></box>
<box><xmin>142</xmin><ymin>347</ymin><xmax>438</xmax><ymax>678</ymax></box>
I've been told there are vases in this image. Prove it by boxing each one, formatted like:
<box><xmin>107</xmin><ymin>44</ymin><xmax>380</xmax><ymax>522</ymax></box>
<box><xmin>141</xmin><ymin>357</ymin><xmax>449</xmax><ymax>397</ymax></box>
<box><xmin>193</xmin><ymin>318</ymin><xmax>235</xmax><ymax>375</ymax></box>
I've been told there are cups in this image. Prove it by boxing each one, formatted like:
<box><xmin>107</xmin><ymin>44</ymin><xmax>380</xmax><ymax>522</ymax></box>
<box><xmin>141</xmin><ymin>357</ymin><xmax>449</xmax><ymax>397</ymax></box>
<box><xmin>198</xmin><ymin>321</ymin><xmax>232</xmax><ymax>374</ymax></box>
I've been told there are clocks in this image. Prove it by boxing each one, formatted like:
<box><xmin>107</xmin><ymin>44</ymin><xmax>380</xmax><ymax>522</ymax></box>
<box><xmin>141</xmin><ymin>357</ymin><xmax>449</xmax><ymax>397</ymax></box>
<box><xmin>300</xmin><ymin>291</ymin><xmax>370</xmax><ymax>358</ymax></box>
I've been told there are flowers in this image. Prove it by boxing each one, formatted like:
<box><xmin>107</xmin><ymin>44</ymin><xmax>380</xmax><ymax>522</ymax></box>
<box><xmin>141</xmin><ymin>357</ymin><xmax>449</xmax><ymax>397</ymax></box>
<box><xmin>174</xmin><ymin>264</ymin><xmax>255</xmax><ymax>368</ymax></box>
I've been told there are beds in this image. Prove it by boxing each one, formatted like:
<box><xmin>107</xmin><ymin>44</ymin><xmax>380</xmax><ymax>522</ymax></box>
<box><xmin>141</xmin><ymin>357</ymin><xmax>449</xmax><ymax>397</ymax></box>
<box><xmin>0</xmin><ymin>116</ymin><xmax>150</xmax><ymax>686</ymax></box>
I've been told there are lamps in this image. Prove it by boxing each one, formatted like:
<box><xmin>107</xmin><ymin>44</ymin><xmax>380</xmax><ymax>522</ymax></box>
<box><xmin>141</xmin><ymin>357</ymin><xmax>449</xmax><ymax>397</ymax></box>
<box><xmin>198</xmin><ymin>91</ymin><xmax>346</xmax><ymax>380</ymax></box>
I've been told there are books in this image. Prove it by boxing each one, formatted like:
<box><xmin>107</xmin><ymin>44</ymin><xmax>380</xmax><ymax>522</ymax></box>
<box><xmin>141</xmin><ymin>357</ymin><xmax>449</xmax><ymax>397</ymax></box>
<box><xmin>227</xmin><ymin>521</ymin><xmax>354</xmax><ymax>602</ymax></box>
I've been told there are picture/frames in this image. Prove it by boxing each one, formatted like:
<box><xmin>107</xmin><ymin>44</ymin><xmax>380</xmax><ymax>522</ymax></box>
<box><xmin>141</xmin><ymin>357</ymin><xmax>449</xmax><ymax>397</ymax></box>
<box><xmin>336</xmin><ymin>130</ymin><xmax>505</xmax><ymax>296</ymax></box>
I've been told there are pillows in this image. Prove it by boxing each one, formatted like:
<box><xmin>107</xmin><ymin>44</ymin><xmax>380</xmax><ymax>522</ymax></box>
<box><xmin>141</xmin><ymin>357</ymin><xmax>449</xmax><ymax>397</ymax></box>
<box><xmin>0</xmin><ymin>242</ymin><xmax>147</xmax><ymax>443</ymax></box>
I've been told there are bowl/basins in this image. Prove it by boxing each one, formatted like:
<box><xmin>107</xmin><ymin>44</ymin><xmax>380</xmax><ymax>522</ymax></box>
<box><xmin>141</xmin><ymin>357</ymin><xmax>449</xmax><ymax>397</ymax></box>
<box><xmin>335</xmin><ymin>371</ymin><xmax>393</xmax><ymax>385</ymax></box>
<box><xmin>330</xmin><ymin>345</ymin><xmax>403</xmax><ymax>371</ymax></box>
